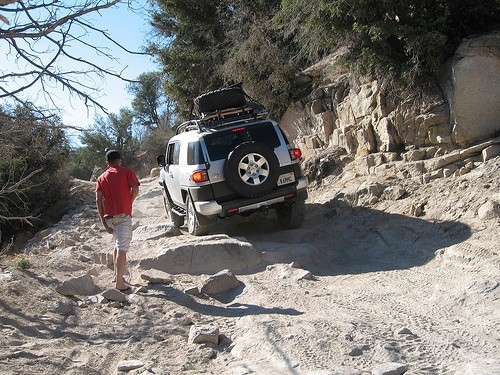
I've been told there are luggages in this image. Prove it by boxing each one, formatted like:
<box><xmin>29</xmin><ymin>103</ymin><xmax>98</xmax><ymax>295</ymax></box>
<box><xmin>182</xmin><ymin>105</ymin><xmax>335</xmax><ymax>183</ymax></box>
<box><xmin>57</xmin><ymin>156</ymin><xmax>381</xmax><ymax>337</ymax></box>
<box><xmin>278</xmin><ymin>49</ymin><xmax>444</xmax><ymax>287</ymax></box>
<box><xmin>194</xmin><ymin>86</ymin><xmax>252</xmax><ymax>124</ymax></box>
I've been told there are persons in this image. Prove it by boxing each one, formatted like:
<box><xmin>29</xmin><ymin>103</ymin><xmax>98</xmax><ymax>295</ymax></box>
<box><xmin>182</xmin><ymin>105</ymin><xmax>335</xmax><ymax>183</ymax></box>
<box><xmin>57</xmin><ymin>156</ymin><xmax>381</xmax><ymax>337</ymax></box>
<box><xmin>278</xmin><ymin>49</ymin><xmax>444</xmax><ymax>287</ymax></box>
<box><xmin>96</xmin><ymin>150</ymin><xmax>140</xmax><ymax>291</ymax></box>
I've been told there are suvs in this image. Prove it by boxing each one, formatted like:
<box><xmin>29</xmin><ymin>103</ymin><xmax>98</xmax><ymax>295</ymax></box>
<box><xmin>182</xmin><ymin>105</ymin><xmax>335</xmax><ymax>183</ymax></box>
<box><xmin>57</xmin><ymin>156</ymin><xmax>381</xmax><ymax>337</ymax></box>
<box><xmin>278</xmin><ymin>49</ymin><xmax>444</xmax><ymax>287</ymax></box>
<box><xmin>156</xmin><ymin>81</ymin><xmax>309</xmax><ymax>236</ymax></box>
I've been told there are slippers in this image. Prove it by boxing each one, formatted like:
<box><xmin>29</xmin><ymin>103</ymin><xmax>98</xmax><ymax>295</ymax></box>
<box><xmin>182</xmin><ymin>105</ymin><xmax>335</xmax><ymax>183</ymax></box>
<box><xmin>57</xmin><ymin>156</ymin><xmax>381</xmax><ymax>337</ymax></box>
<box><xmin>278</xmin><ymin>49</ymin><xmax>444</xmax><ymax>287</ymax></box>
<box><xmin>112</xmin><ymin>277</ymin><xmax>125</xmax><ymax>283</ymax></box>
<box><xmin>118</xmin><ymin>285</ymin><xmax>130</xmax><ymax>291</ymax></box>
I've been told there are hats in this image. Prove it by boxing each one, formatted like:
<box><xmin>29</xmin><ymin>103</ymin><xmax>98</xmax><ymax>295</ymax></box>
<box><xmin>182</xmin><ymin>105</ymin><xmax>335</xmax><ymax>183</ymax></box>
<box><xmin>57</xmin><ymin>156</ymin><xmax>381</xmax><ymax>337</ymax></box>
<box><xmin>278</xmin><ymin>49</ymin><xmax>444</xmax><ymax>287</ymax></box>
<box><xmin>106</xmin><ymin>150</ymin><xmax>120</xmax><ymax>160</ymax></box>
